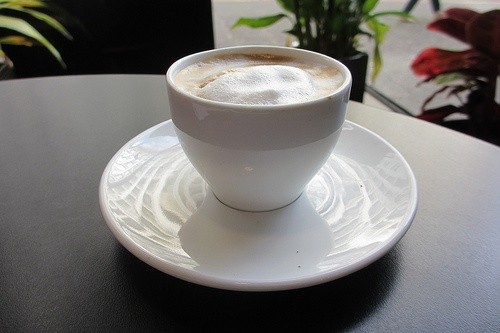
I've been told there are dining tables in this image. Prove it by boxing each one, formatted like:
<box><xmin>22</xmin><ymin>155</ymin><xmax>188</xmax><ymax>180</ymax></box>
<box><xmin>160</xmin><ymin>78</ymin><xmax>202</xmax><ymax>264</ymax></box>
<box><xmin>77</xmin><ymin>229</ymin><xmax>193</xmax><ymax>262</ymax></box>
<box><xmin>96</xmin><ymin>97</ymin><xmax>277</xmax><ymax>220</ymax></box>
<box><xmin>0</xmin><ymin>74</ymin><xmax>500</xmax><ymax>333</ymax></box>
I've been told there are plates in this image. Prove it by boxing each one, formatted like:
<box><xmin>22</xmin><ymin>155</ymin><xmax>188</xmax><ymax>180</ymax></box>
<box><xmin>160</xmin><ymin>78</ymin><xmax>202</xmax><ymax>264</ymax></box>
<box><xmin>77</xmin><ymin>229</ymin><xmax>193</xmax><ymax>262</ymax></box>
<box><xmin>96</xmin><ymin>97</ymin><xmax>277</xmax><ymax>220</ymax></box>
<box><xmin>98</xmin><ymin>119</ymin><xmax>418</xmax><ymax>292</ymax></box>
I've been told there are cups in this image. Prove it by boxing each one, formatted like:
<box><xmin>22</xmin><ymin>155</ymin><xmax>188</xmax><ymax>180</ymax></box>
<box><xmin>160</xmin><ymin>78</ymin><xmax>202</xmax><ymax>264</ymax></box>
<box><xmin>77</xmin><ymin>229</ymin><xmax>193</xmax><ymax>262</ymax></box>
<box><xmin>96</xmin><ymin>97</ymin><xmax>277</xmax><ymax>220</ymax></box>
<box><xmin>165</xmin><ymin>45</ymin><xmax>353</xmax><ymax>213</ymax></box>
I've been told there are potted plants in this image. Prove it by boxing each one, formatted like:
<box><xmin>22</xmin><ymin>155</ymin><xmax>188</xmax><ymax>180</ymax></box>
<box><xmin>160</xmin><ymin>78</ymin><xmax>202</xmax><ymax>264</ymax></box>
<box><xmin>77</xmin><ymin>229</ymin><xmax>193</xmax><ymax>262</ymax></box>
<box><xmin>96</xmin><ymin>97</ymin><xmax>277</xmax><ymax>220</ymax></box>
<box><xmin>413</xmin><ymin>7</ymin><xmax>500</xmax><ymax>146</ymax></box>
<box><xmin>231</xmin><ymin>0</ymin><xmax>416</xmax><ymax>105</ymax></box>
